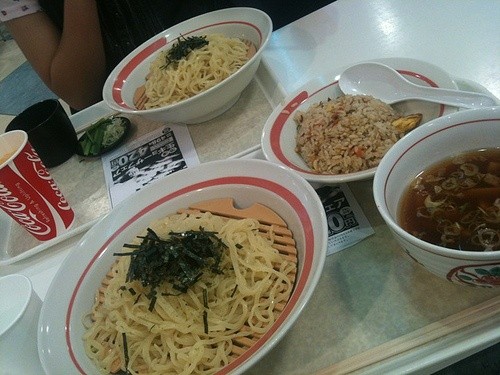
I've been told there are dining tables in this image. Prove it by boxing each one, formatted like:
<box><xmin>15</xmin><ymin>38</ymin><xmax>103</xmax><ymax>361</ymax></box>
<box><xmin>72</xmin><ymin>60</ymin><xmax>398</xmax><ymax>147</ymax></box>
<box><xmin>0</xmin><ymin>0</ymin><xmax>500</xmax><ymax>375</ymax></box>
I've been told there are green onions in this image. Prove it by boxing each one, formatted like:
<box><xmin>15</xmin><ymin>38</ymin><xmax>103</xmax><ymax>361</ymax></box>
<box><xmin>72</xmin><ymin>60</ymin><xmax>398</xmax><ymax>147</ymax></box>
<box><xmin>406</xmin><ymin>152</ymin><xmax>500</xmax><ymax>252</ymax></box>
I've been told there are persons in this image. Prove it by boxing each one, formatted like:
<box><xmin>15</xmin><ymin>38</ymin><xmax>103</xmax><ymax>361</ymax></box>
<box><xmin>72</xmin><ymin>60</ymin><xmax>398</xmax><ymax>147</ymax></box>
<box><xmin>0</xmin><ymin>0</ymin><xmax>334</xmax><ymax>114</ymax></box>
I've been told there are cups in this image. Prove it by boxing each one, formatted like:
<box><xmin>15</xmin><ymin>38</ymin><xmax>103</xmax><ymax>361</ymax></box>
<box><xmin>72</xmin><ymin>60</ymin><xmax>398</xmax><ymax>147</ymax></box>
<box><xmin>0</xmin><ymin>130</ymin><xmax>75</xmax><ymax>241</ymax></box>
<box><xmin>0</xmin><ymin>274</ymin><xmax>46</xmax><ymax>375</ymax></box>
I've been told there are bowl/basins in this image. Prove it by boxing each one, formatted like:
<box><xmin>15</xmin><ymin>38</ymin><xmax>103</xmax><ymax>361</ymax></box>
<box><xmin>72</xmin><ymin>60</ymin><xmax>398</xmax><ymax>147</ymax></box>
<box><xmin>373</xmin><ymin>109</ymin><xmax>499</xmax><ymax>290</ymax></box>
<box><xmin>261</xmin><ymin>56</ymin><xmax>461</xmax><ymax>182</ymax></box>
<box><xmin>5</xmin><ymin>98</ymin><xmax>77</xmax><ymax>168</ymax></box>
<box><xmin>102</xmin><ymin>7</ymin><xmax>273</xmax><ymax>124</ymax></box>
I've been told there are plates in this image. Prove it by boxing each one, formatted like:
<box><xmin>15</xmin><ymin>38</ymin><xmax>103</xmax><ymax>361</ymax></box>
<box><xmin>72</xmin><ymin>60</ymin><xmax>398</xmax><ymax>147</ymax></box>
<box><xmin>36</xmin><ymin>158</ymin><xmax>328</xmax><ymax>375</ymax></box>
<box><xmin>75</xmin><ymin>116</ymin><xmax>131</xmax><ymax>157</ymax></box>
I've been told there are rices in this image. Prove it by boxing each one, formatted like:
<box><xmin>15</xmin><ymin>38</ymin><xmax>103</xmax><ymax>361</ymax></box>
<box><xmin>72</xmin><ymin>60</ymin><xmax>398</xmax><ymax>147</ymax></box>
<box><xmin>294</xmin><ymin>94</ymin><xmax>423</xmax><ymax>175</ymax></box>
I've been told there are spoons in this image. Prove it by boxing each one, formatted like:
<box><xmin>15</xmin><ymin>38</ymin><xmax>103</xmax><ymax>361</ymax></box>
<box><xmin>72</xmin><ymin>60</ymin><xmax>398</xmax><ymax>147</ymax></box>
<box><xmin>339</xmin><ymin>62</ymin><xmax>497</xmax><ymax>108</ymax></box>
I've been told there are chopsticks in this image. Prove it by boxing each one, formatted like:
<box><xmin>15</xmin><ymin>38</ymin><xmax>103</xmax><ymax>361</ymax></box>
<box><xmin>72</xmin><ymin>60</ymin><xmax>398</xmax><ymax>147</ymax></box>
<box><xmin>316</xmin><ymin>296</ymin><xmax>500</xmax><ymax>375</ymax></box>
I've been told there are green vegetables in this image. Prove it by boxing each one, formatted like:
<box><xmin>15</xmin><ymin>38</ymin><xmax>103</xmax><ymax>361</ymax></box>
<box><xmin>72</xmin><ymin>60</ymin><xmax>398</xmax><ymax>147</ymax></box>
<box><xmin>78</xmin><ymin>114</ymin><xmax>127</xmax><ymax>156</ymax></box>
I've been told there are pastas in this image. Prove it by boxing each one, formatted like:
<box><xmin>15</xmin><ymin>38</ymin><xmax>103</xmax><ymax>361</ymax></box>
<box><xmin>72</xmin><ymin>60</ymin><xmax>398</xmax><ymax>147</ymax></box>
<box><xmin>145</xmin><ymin>34</ymin><xmax>250</xmax><ymax>109</ymax></box>
<box><xmin>82</xmin><ymin>212</ymin><xmax>293</xmax><ymax>375</ymax></box>
